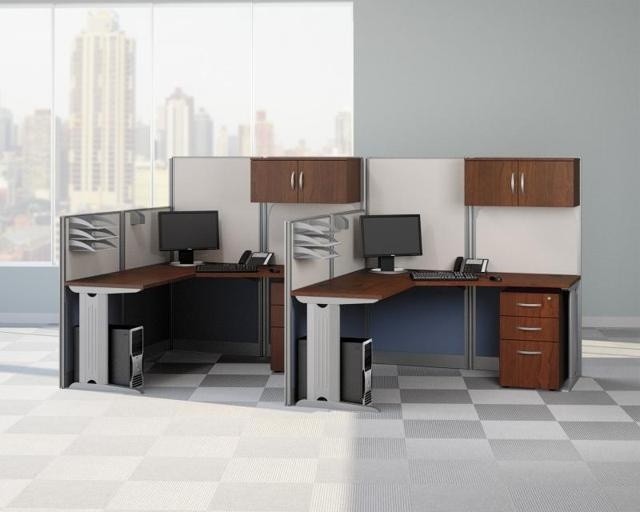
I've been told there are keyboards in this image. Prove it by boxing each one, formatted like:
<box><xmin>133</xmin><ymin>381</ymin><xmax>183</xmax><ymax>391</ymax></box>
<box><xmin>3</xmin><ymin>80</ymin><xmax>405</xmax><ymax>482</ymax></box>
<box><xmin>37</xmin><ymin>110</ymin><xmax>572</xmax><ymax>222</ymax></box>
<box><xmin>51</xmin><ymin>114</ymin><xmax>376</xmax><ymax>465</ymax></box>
<box><xmin>195</xmin><ymin>264</ymin><xmax>257</xmax><ymax>273</ymax></box>
<box><xmin>411</xmin><ymin>271</ymin><xmax>479</xmax><ymax>281</ymax></box>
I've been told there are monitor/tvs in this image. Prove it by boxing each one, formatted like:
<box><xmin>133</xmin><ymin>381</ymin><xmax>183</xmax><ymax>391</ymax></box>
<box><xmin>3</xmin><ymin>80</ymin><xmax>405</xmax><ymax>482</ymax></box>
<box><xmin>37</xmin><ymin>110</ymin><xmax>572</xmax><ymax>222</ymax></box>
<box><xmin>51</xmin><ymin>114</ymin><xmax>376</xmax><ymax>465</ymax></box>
<box><xmin>159</xmin><ymin>211</ymin><xmax>219</xmax><ymax>267</ymax></box>
<box><xmin>360</xmin><ymin>214</ymin><xmax>422</xmax><ymax>275</ymax></box>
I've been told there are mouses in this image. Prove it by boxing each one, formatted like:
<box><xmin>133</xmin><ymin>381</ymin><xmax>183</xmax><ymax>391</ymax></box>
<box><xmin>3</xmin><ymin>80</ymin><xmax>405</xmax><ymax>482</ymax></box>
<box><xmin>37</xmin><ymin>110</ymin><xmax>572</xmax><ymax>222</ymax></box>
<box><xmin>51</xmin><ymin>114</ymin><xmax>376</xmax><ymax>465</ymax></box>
<box><xmin>490</xmin><ymin>275</ymin><xmax>502</xmax><ymax>281</ymax></box>
<box><xmin>270</xmin><ymin>266</ymin><xmax>280</xmax><ymax>273</ymax></box>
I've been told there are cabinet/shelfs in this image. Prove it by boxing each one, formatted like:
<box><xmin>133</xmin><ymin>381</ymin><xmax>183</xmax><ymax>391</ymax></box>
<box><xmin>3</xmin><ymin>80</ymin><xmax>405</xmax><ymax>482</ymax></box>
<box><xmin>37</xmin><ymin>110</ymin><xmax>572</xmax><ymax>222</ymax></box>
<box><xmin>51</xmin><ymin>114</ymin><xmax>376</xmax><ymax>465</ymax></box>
<box><xmin>465</xmin><ymin>157</ymin><xmax>580</xmax><ymax>206</ymax></box>
<box><xmin>270</xmin><ymin>282</ymin><xmax>283</xmax><ymax>372</ymax></box>
<box><xmin>499</xmin><ymin>291</ymin><xmax>569</xmax><ymax>390</ymax></box>
<box><xmin>250</xmin><ymin>157</ymin><xmax>360</xmax><ymax>204</ymax></box>
<box><xmin>69</xmin><ymin>215</ymin><xmax>119</xmax><ymax>252</ymax></box>
<box><xmin>294</xmin><ymin>221</ymin><xmax>341</xmax><ymax>260</ymax></box>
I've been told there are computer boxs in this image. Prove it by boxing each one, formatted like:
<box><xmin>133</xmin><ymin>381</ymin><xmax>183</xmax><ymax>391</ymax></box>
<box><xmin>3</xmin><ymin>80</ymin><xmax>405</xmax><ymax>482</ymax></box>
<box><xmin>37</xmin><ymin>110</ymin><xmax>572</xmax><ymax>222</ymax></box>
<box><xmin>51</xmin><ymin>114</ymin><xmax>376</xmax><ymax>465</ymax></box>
<box><xmin>74</xmin><ymin>323</ymin><xmax>145</xmax><ymax>390</ymax></box>
<box><xmin>298</xmin><ymin>335</ymin><xmax>373</xmax><ymax>405</ymax></box>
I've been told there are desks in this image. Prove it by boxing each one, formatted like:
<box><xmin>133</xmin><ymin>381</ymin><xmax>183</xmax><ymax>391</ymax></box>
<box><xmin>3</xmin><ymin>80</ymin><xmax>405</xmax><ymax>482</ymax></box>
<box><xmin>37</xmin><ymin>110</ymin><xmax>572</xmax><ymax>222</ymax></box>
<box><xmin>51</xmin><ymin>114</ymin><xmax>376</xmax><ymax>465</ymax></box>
<box><xmin>66</xmin><ymin>262</ymin><xmax>283</xmax><ymax>386</ymax></box>
<box><xmin>292</xmin><ymin>267</ymin><xmax>581</xmax><ymax>402</ymax></box>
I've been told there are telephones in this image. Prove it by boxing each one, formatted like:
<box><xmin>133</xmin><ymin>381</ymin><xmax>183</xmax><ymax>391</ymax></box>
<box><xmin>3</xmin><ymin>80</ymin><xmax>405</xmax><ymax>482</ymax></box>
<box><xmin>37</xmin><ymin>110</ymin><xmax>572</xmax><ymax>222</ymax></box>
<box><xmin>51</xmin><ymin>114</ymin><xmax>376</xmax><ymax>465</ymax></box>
<box><xmin>238</xmin><ymin>250</ymin><xmax>274</xmax><ymax>265</ymax></box>
<box><xmin>453</xmin><ymin>257</ymin><xmax>489</xmax><ymax>276</ymax></box>
